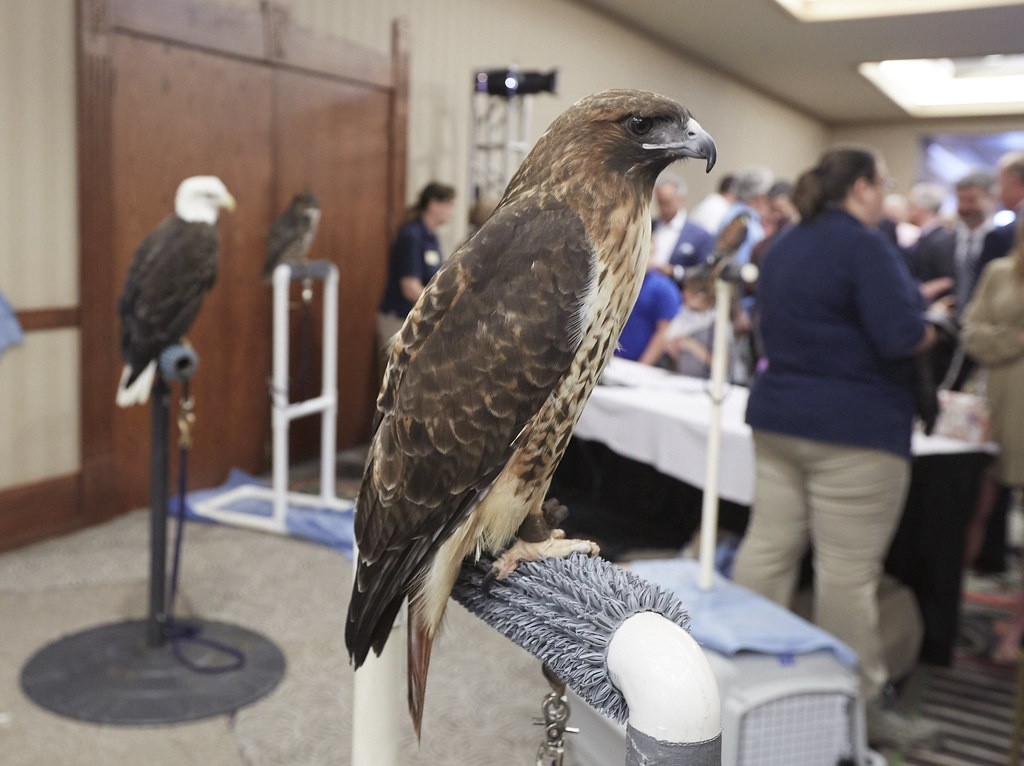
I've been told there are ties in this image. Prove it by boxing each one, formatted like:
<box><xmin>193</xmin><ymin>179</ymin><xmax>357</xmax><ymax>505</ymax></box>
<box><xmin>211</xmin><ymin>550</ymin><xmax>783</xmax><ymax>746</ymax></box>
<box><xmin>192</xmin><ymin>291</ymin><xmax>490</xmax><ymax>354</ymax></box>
<box><xmin>961</xmin><ymin>233</ymin><xmax>973</xmax><ymax>278</ymax></box>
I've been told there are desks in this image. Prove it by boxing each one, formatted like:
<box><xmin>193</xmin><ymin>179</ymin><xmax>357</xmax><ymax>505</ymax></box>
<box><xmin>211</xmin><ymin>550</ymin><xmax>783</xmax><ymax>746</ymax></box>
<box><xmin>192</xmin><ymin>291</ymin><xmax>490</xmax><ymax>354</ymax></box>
<box><xmin>564</xmin><ymin>355</ymin><xmax>1003</xmax><ymax>670</ymax></box>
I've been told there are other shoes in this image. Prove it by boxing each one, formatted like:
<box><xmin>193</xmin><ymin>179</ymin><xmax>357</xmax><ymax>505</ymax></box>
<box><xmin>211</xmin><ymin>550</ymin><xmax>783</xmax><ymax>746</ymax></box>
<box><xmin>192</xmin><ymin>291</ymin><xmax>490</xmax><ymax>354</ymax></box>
<box><xmin>963</xmin><ymin>567</ymin><xmax>1002</xmax><ymax>594</ymax></box>
<box><xmin>860</xmin><ymin>703</ymin><xmax>936</xmax><ymax>746</ymax></box>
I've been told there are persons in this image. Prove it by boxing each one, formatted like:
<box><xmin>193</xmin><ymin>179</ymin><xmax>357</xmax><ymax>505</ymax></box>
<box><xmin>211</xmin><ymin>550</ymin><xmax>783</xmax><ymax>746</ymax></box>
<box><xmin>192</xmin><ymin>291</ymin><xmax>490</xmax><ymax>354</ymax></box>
<box><xmin>729</xmin><ymin>147</ymin><xmax>955</xmax><ymax>748</ymax></box>
<box><xmin>374</xmin><ymin>181</ymin><xmax>458</xmax><ymax>366</ymax></box>
<box><xmin>544</xmin><ymin>150</ymin><xmax>1024</xmax><ymax>667</ymax></box>
